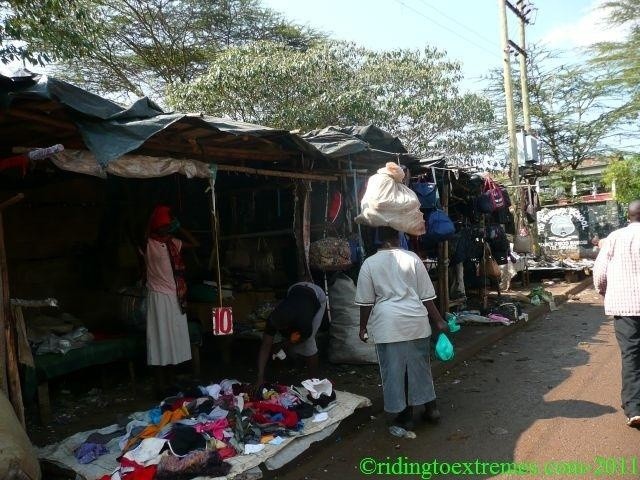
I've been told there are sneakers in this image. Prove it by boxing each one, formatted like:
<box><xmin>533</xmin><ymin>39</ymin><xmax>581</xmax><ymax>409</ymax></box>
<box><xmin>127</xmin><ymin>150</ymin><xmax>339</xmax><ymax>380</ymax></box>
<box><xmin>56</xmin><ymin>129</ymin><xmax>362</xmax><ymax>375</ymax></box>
<box><xmin>625</xmin><ymin>416</ymin><xmax>640</xmax><ymax>425</ymax></box>
<box><xmin>420</xmin><ymin>407</ymin><xmax>442</xmax><ymax>424</ymax></box>
<box><xmin>392</xmin><ymin>417</ymin><xmax>416</xmax><ymax>431</ymax></box>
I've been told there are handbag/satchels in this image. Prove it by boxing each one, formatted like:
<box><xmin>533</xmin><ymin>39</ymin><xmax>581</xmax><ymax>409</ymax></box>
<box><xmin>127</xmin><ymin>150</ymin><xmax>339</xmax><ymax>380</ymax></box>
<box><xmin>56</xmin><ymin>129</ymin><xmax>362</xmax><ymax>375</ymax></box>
<box><xmin>253</xmin><ymin>237</ymin><xmax>282</xmax><ymax>271</ymax></box>
<box><xmin>411</xmin><ymin>176</ymin><xmax>437</xmax><ymax>209</ymax></box>
<box><xmin>480</xmin><ymin>242</ymin><xmax>501</xmax><ymax>278</ymax></box>
<box><xmin>480</xmin><ymin>177</ymin><xmax>505</xmax><ymax>212</ymax></box>
<box><xmin>427</xmin><ymin>210</ymin><xmax>456</xmax><ymax>241</ymax></box>
<box><xmin>488</xmin><ymin>221</ymin><xmax>508</xmax><ymax>264</ymax></box>
<box><xmin>311</xmin><ymin>221</ymin><xmax>353</xmax><ymax>269</ymax></box>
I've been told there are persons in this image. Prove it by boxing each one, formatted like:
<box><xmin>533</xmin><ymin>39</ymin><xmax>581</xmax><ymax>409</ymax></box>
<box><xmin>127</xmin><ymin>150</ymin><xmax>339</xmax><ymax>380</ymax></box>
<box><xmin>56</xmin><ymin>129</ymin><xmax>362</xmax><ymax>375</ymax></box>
<box><xmin>242</xmin><ymin>281</ymin><xmax>327</xmax><ymax>399</ymax></box>
<box><xmin>128</xmin><ymin>204</ymin><xmax>202</xmax><ymax>393</ymax></box>
<box><xmin>592</xmin><ymin>200</ymin><xmax>640</xmax><ymax>428</ymax></box>
<box><xmin>353</xmin><ymin>225</ymin><xmax>451</xmax><ymax>430</ymax></box>
<box><xmin>593</xmin><ymin>237</ymin><xmax>606</xmax><ymax>248</ymax></box>
